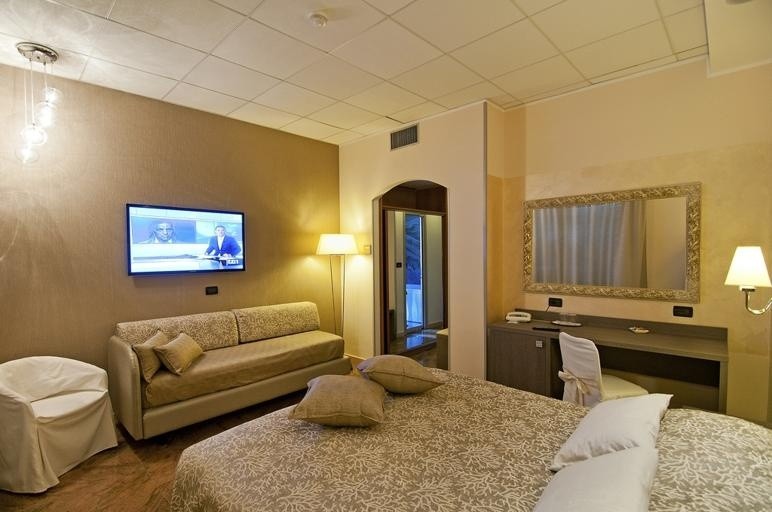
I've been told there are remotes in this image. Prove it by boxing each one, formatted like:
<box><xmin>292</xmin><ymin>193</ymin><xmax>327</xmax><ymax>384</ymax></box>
<box><xmin>552</xmin><ymin>320</ymin><xmax>582</xmax><ymax>327</ymax></box>
<box><xmin>531</xmin><ymin>326</ymin><xmax>564</xmax><ymax>332</ymax></box>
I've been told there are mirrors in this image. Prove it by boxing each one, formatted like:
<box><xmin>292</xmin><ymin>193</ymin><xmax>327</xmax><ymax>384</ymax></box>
<box><xmin>522</xmin><ymin>181</ymin><xmax>702</xmax><ymax>300</ymax></box>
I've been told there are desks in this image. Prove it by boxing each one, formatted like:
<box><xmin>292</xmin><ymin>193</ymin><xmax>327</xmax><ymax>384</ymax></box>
<box><xmin>485</xmin><ymin>318</ymin><xmax>729</xmax><ymax>414</ymax></box>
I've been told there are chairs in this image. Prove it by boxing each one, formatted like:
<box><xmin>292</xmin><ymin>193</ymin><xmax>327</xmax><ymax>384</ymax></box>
<box><xmin>556</xmin><ymin>331</ymin><xmax>649</xmax><ymax>407</ymax></box>
<box><xmin>0</xmin><ymin>355</ymin><xmax>119</xmax><ymax>496</ymax></box>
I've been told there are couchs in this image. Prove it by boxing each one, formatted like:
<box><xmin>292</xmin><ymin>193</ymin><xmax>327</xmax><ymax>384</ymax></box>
<box><xmin>106</xmin><ymin>300</ymin><xmax>354</xmax><ymax>441</ymax></box>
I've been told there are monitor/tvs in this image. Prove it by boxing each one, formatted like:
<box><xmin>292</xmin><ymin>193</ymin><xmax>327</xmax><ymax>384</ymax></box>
<box><xmin>125</xmin><ymin>202</ymin><xmax>246</xmax><ymax>276</ymax></box>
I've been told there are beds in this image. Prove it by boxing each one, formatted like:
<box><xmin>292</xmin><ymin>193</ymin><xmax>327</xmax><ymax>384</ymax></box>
<box><xmin>170</xmin><ymin>366</ymin><xmax>772</xmax><ymax>512</ymax></box>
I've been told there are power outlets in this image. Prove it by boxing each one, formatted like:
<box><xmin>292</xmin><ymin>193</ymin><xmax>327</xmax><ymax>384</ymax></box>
<box><xmin>548</xmin><ymin>297</ymin><xmax>562</xmax><ymax>307</ymax></box>
<box><xmin>673</xmin><ymin>306</ymin><xmax>693</xmax><ymax>317</ymax></box>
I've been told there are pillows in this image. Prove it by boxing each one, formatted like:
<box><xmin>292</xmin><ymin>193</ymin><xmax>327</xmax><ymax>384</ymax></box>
<box><xmin>532</xmin><ymin>445</ymin><xmax>659</xmax><ymax>512</ymax></box>
<box><xmin>356</xmin><ymin>354</ymin><xmax>446</xmax><ymax>396</ymax></box>
<box><xmin>132</xmin><ymin>331</ymin><xmax>171</xmax><ymax>384</ymax></box>
<box><xmin>548</xmin><ymin>391</ymin><xmax>673</xmax><ymax>473</ymax></box>
<box><xmin>152</xmin><ymin>330</ymin><xmax>204</xmax><ymax>377</ymax></box>
<box><xmin>287</xmin><ymin>374</ymin><xmax>389</xmax><ymax>428</ymax></box>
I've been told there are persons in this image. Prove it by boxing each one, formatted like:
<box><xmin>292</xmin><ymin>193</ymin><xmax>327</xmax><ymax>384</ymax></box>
<box><xmin>205</xmin><ymin>225</ymin><xmax>242</xmax><ymax>260</ymax></box>
<box><xmin>138</xmin><ymin>220</ymin><xmax>188</xmax><ymax>244</ymax></box>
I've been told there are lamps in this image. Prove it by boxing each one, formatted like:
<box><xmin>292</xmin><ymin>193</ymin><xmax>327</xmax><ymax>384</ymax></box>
<box><xmin>315</xmin><ymin>232</ymin><xmax>357</xmax><ymax>337</ymax></box>
<box><xmin>15</xmin><ymin>42</ymin><xmax>64</xmax><ymax>165</ymax></box>
<box><xmin>724</xmin><ymin>246</ymin><xmax>772</xmax><ymax>316</ymax></box>
